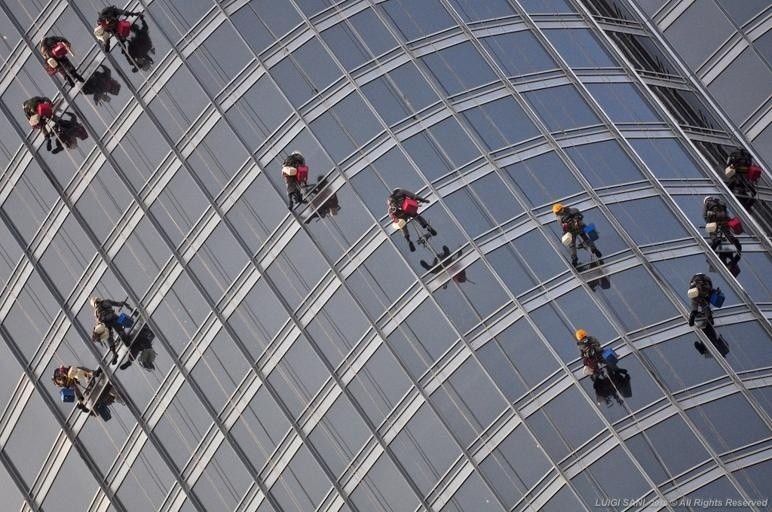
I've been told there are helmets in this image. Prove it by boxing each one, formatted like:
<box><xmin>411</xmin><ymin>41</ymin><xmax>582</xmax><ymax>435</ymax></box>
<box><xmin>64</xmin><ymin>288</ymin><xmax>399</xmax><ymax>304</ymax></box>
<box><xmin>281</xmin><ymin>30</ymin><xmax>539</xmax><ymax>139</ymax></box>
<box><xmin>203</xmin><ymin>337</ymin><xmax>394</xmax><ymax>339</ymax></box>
<box><xmin>576</xmin><ymin>330</ymin><xmax>587</xmax><ymax>340</ymax></box>
<box><xmin>291</xmin><ymin>151</ymin><xmax>302</xmax><ymax>155</ymax></box>
<box><xmin>90</xmin><ymin>297</ymin><xmax>101</xmax><ymax>307</ymax></box>
<box><xmin>553</xmin><ymin>204</ymin><xmax>562</xmax><ymax>213</ymax></box>
<box><xmin>704</xmin><ymin>196</ymin><xmax>711</xmax><ymax>204</ymax></box>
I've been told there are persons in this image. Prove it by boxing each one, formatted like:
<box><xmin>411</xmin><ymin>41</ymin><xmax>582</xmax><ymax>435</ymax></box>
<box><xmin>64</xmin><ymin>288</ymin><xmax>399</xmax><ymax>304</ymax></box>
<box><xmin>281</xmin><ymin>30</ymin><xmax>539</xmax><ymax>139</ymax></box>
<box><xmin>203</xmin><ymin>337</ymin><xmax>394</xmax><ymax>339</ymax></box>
<box><xmin>693</xmin><ymin>320</ymin><xmax>732</xmax><ymax>362</ymax></box>
<box><xmin>550</xmin><ymin>201</ymin><xmax>602</xmax><ymax>268</ymax></box>
<box><xmin>122</xmin><ymin>15</ymin><xmax>154</xmax><ymax>75</ymax></box>
<box><xmin>74</xmin><ymin>373</ymin><xmax>114</xmax><ymax>423</ymax></box>
<box><xmin>280</xmin><ymin>151</ymin><xmax>308</xmax><ymax>212</ymax></box>
<box><xmin>121</xmin><ymin>321</ymin><xmax>157</xmax><ymax>372</ymax></box>
<box><xmin>49</xmin><ymin>363</ymin><xmax>99</xmax><ymax>405</ymax></box>
<box><xmin>726</xmin><ymin>146</ymin><xmax>761</xmax><ymax>197</ymax></box>
<box><xmin>88</xmin><ymin>293</ymin><xmax>133</xmax><ymax>365</ymax></box>
<box><xmin>592</xmin><ymin>373</ymin><xmax>633</xmax><ymax>406</ymax></box>
<box><xmin>19</xmin><ymin>95</ymin><xmax>58</xmax><ymax>151</ymax></box>
<box><xmin>575</xmin><ymin>329</ymin><xmax>627</xmax><ymax>391</ymax></box>
<box><xmin>50</xmin><ymin>107</ymin><xmax>90</xmax><ymax>154</ymax></box>
<box><xmin>419</xmin><ymin>245</ymin><xmax>468</xmax><ymax>290</ymax></box>
<box><xmin>80</xmin><ymin>64</ymin><xmax>123</xmax><ymax>105</ymax></box>
<box><xmin>38</xmin><ymin>36</ymin><xmax>88</xmax><ymax>89</ymax></box>
<box><xmin>304</xmin><ymin>177</ymin><xmax>339</xmax><ymax>223</ymax></box>
<box><xmin>95</xmin><ymin>4</ymin><xmax>147</xmax><ymax>53</ymax></box>
<box><xmin>701</xmin><ymin>194</ymin><xmax>742</xmax><ymax>257</ymax></box>
<box><xmin>388</xmin><ymin>186</ymin><xmax>436</xmax><ymax>251</ymax></box>
<box><xmin>687</xmin><ymin>269</ymin><xmax>717</xmax><ymax>327</ymax></box>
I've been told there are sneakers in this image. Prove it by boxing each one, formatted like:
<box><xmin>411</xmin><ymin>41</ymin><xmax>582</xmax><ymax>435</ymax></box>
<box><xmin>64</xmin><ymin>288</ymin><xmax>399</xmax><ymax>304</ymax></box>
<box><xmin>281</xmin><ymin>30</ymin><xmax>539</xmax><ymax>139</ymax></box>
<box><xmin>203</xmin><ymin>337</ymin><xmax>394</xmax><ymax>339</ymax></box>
<box><xmin>595</xmin><ymin>250</ymin><xmax>601</xmax><ymax>258</ymax></box>
<box><xmin>409</xmin><ymin>242</ymin><xmax>415</xmax><ymax>251</ymax></box>
<box><xmin>95</xmin><ymin>367</ymin><xmax>102</xmax><ymax>376</ymax></box>
<box><xmin>733</xmin><ymin>238</ymin><xmax>741</xmax><ymax>251</ymax></box>
<box><xmin>47</xmin><ymin>139</ymin><xmax>51</xmax><ymax>150</ymax></box>
<box><xmin>614</xmin><ymin>368</ymin><xmax>627</xmax><ymax>373</ymax></box>
<box><xmin>105</xmin><ymin>39</ymin><xmax>110</xmax><ymax>52</ymax></box>
<box><xmin>428</xmin><ymin>227</ymin><xmax>436</xmax><ymax>235</ymax></box>
<box><xmin>572</xmin><ymin>256</ymin><xmax>578</xmax><ymax>265</ymax></box>
<box><xmin>73</xmin><ymin>73</ymin><xmax>84</xmax><ymax>82</ymax></box>
<box><xmin>112</xmin><ymin>354</ymin><xmax>118</xmax><ymax>364</ymax></box>
<box><xmin>66</xmin><ymin>76</ymin><xmax>75</xmax><ymax>87</ymax></box>
<box><xmin>689</xmin><ymin>313</ymin><xmax>695</xmax><ymax>326</ymax></box>
<box><xmin>121</xmin><ymin>334</ymin><xmax>130</xmax><ymax>346</ymax></box>
<box><xmin>121</xmin><ymin>45</ymin><xmax>126</xmax><ymax>54</ymax></box>
<box><xmin>77</xmin><ymin>403</ymin><xmax>88</xmax><ymax>412</ymax></box>
<box><xmin>288</xmin><ymin>202</ymin><xmax>293</xmax><ymax>209</ymax></box>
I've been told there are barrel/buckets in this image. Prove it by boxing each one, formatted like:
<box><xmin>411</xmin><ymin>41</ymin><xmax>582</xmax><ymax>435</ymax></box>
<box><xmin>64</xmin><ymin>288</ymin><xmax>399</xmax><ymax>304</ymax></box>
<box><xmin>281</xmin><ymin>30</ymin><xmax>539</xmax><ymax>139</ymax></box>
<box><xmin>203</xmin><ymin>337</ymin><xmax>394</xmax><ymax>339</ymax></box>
<box><xmin>729</xmin><ymin>218</ymin><xmax>744</xmax><ymax>234</ymax></box>
<box><xmin>601</xmin><ymin>348</ymin><xmax>618</xmax><ymax>369</ymax></box>
<box><xmin>117</xmin><ymin>18</ymin><xmax>131</xmax><ymax>38</ymax></box>
<box><xmin>583</xmin><ymin>365</ymin><xmax>594</xmax><ymax>375</ymax></box>
<box><xmin>297</xmin><ymin>166</ymin><xmax>308</xmax><ymax>182</ymax></box>
<box><xmin>583</xmin><ymin>222</ymin><xmax>599</xmax><ymax>242</ymax></box>
<box><xmin>710</xmin><ymin>292</ymin><xmax>725</xmax><ymax>307</ymax></box>
<box><xmin>77</xmin><ymin>369</ymin><xmax>85</xmax><ymax>382</ymax></box>
<box><xmin>725</xmin><ymin>166</ymin><xmax>735</xmax><ymax>179</ymax></box>
<box><xmin>47</xmin><ymin>56</ymin><xmax>58</xmax><ymax>70</ymax></box>
<box><xmin>94</xmin><ymin>323</ymin><xmax>105</xmax><ymax>336</ymax></box>
<box><xmin>392</xmin><ymin>218</ymin><xmax>407</xmax><ymax>230</ymax></box>
<box><xmin>687</xmin><ymin>287</ymin><xmax>699</xmax><ymax>299</ymax></box>
<box><xmin>52</xmin><ymin>44</ymin><xmax>66</xmax><ymax>58</ymax></box>
<box><xmin>37</xmin><ymin>103</ymin><xmax>52</xmax><ymax>117</ymax></box>
<box><xmin>67</xmin><ymin>366</ymin><xmax>77</xmax><ymax>381</ymax></box>
<box><xmin>116</xmin><ymin>313</ymin><xmax>128</xmax><ymax>325</ymax></box>
<box><xmin>59</xmin><ymin>388</ymin><xmax>75</xmax><ymax>402</ymax></box>
<box><xmin>401</xmin><ymin>197</ymin><xmax>418</xmax><ymax>217</ymax></box>
<box><xmin>29</xmin><ymin>114</ymin><xmax>39</xmax><ymax>127</ymax></box>
<box><xmin>99</xmin><ymin>328</ymin><xmax>110</xmax><ymax>340</ymax></box>
<box><xmin>281</xmin><ymin>165</ymin><xmax>297</xmax><ymax>176</ymax></box>
<box><xmin>748</xmin><ymin>165</ymin><xmax>761</xmax><ymax>182</ymax></box>
<box><xmin>705</xmin><ymin>222</ymin><xmax>717</xmax><ymax>233</ymax></box>
<box><xmin>94</xmin><ymin>25</ymin><xmax>105</xmax><ymax>36</ymax></box>
<box><xmin>561</xmin><ymin>231</ymin><xmax>572</xmax><ymax>246</ymax></box>
